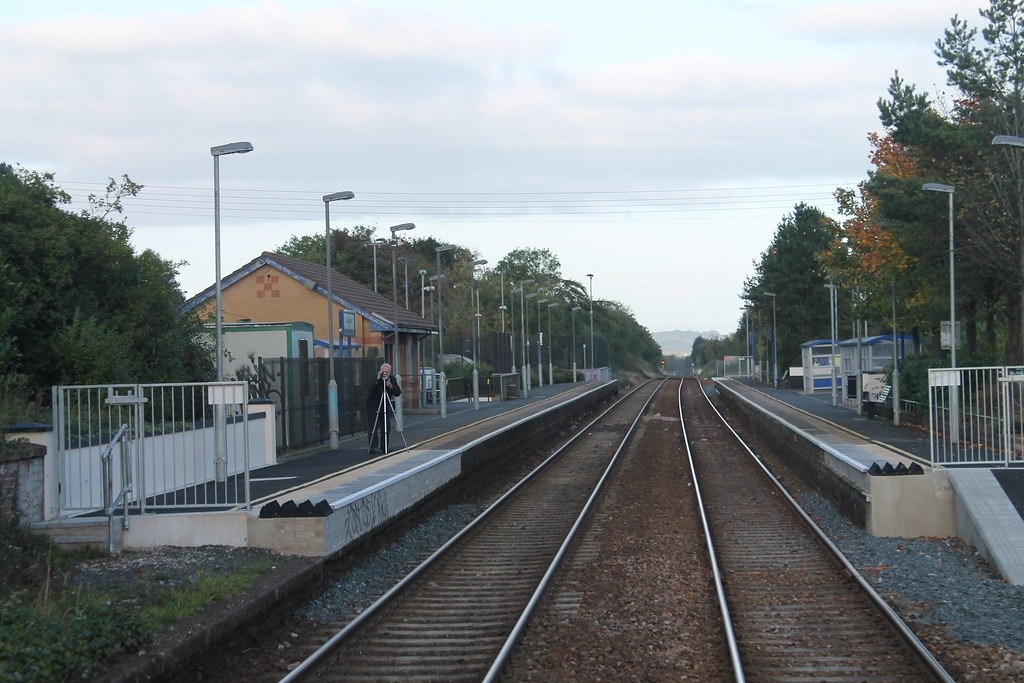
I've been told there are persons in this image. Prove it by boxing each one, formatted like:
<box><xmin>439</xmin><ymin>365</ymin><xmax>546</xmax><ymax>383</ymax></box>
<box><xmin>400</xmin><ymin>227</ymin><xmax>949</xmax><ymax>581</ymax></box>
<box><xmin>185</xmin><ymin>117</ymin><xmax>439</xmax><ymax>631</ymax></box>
<box><xmin>366</xmin><ymin>363</ymin><xmax>401</xmax><ymax>454</ymax></box>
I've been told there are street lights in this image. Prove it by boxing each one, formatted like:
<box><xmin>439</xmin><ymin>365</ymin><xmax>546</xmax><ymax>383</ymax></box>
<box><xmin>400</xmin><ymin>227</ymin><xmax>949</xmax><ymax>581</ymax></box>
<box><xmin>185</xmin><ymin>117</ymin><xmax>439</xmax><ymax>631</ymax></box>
<box><xmin>824</xmin><ymin>284</ymin><xmax>839</xmax><ymax>404</ymax></box>
<box><xmin>739</xmin><ymin>305</ymin><xmax>756</xmax><ymax>380</ymax></box>
<box><xmin>763</xmin><ymin>292</ymin><xmax>777</xmax><ymax>387</ymax></box>
<box><xmin>471</xmin><ymin>259</ymin><xmax>487</xmax><ymax>409</ymax></box>
<box><xmin>436</xmin><ymin>244</ymin><xmax>456</xmax><ymax>417</ymax></box>
<box><xmin>211</xmin><ymin>141</ymin><xmax>253</xmax><ymax>483</ymax></box>
<box><xmin>499</xmin><ymin>270</ymin><xmax>594</xmax><ymax>398</ymax></box>
<box><xmin>923</xmin><ymin>182</ymin><xmax>959</xmax><ymax>443</ymax></box>
<box><xmin>391</xmin><ymin>222</ymin><xmax>416</xmax><ymax>432</ymax></box>
<box><xmin>323</xmin><ymin>191</ymin><xmax>355</xmax><ymax>449</ymax></box>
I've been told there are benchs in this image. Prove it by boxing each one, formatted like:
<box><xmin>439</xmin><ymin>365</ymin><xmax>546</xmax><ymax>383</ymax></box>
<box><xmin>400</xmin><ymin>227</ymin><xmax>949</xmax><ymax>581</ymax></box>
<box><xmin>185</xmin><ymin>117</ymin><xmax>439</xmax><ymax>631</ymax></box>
<box><xmin>862</xmin><ymin>386</ymin><xmax>892</xmax><ymax>419</ymax></box>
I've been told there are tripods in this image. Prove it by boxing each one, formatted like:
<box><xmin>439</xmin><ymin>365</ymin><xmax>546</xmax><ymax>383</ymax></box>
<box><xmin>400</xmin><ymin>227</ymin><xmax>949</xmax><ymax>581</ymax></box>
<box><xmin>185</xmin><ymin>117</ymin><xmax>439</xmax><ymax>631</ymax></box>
<box><xmin>367</xmin><ymin>377</ymin><xmax>409</xmax><ymax>454</ymax></box>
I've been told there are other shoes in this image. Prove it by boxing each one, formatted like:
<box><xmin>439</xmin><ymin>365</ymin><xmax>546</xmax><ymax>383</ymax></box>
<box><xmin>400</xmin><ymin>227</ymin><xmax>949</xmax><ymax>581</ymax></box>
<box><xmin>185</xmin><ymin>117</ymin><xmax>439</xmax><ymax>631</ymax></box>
<box><xmin>372</xmin><ymin>448</ymin><xmax>392</xmax><ymax>454</ymax></box>
